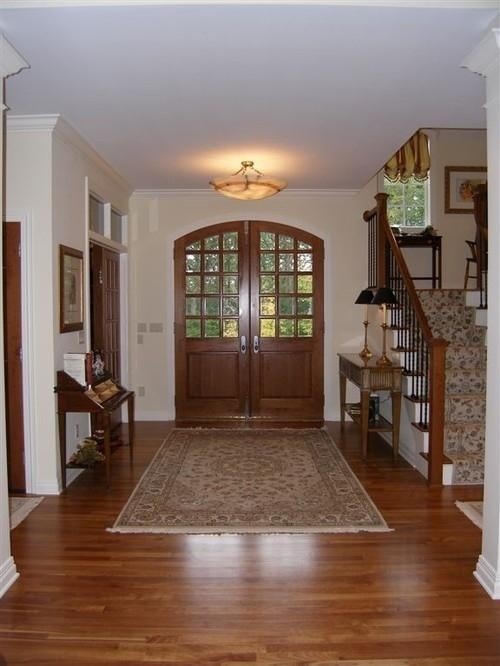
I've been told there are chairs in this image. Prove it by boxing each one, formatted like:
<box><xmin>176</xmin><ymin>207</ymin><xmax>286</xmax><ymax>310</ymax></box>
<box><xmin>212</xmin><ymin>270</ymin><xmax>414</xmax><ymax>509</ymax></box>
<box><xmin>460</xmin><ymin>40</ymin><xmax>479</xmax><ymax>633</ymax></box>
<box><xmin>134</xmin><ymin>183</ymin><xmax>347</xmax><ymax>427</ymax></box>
<box><xmin>463</xmin><ymin>240</ymin><xmax>482</xmax><ymax>290</ymax></box>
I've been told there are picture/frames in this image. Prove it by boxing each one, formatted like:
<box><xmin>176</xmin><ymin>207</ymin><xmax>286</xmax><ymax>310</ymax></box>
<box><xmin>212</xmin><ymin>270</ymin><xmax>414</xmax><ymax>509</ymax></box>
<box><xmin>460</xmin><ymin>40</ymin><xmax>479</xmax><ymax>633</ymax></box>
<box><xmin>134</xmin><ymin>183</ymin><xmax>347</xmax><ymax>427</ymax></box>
<box><xmin>445</xmin><ymin>165</ymin><xmax>488</xmax><ymax>214</ymax></box>
<box><xmin>58</xmin><ymin>243</ymin><xmax>86</xmax><ymax>333</ymax></box>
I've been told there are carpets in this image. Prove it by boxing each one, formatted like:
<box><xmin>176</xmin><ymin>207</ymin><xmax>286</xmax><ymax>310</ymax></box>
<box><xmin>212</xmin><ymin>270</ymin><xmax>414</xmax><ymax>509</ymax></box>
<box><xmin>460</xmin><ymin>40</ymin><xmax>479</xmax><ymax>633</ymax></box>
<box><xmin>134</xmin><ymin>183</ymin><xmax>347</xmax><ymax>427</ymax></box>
<box><xmin>105</xmin><ymin>428</ymin><xmax>397</xmax><ymax>536</ymax></box>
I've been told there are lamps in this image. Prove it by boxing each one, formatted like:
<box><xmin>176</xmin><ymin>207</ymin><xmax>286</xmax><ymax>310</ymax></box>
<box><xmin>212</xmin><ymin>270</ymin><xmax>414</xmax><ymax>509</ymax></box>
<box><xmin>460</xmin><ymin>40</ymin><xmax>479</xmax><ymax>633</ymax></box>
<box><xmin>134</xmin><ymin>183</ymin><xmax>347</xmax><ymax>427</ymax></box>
<box><xmin>353</xmin><ymin>286</ymin><xmax>399</xmax><ymax>366</ymax></box>
<box><xmin>208</xmin><ymin>161</ymin><xmax>289</xmax><ymax>201</ymax></box>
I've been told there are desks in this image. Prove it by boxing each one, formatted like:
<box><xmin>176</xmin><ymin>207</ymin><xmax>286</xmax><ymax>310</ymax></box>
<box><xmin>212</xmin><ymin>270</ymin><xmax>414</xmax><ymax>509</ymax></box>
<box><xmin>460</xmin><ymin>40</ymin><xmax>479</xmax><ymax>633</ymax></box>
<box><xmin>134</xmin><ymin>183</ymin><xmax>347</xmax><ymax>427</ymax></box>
<box><xmin>336</xmin><ymin>353</ymin><xmax>405</xmax><ymax>466</ymax></box>
<box><xmin>386</xmin><ymin>234</ymin><xmax>443</xmax><ymax>290</ymax></box>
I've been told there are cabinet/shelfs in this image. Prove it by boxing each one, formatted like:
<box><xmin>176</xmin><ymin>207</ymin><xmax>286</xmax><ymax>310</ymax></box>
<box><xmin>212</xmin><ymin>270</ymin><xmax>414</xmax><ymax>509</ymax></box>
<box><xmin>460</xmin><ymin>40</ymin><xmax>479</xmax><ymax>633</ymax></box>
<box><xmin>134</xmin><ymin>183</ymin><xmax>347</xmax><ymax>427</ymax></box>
<box><xmin>56</xmin><ymin>365</ymin><xmax>135</xmax><ymax>489</ymax></box>
